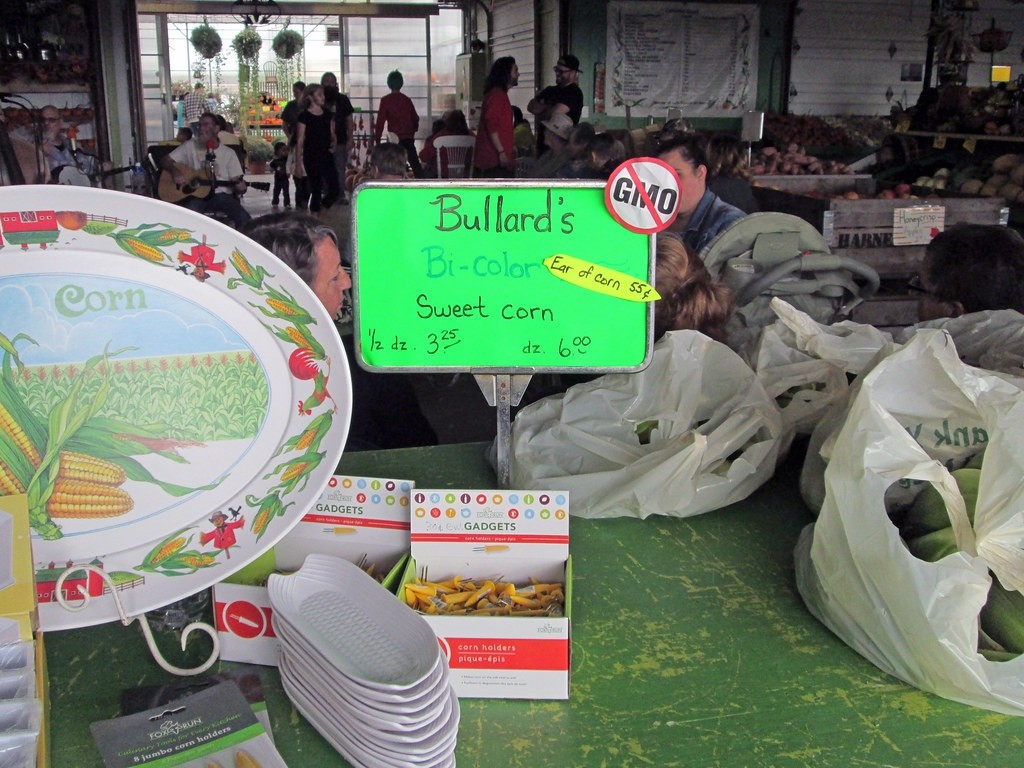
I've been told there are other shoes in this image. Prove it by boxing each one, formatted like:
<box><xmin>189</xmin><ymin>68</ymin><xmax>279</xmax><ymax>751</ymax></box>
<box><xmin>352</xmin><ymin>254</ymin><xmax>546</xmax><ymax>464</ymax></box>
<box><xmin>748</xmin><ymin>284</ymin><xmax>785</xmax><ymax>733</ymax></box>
<box><xmin>272</xmin><ymin>204</ymin><xmax>278</xmax><ymax>208</ymax></box>
<box><xmin>286</xmin><ymin>204</ymin><xmax>291</xmax><ymax>208</ymax></box>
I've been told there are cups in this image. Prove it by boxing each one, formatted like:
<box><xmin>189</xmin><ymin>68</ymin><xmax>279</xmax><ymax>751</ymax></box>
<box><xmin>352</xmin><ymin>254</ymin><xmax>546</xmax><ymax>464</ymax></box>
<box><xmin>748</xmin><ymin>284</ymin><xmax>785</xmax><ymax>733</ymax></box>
<box><xmin>145</xmin><ymin>587</ymin><xmax>210</xmax><ymax>634</ymax></box>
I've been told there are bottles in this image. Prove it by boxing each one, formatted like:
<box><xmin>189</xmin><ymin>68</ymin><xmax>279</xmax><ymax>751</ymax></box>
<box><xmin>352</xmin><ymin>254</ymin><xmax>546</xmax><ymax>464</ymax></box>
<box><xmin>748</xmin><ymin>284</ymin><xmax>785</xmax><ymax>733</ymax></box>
<box><xmin>132</xmin><ymin>162</ymin><xmax>146</xmax><ymax>196</ymax></box>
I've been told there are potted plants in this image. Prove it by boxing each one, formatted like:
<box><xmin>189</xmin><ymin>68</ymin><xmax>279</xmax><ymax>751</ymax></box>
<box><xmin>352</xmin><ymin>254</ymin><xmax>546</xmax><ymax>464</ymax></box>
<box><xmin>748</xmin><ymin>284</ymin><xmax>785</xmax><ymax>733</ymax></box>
<box><xmin>271</xmin><ymin>30</ymin><xmax>304</xmax><ymax>96</ymax></box>
<box><xmin>228</xmin><ymin>28</ymin><xmax>262</xmax><ymax>138</ymax></box>
<box><xmin>189</xmin><ymin>25</ymin><xmax>227</xmax><ymax>96</ymax></box>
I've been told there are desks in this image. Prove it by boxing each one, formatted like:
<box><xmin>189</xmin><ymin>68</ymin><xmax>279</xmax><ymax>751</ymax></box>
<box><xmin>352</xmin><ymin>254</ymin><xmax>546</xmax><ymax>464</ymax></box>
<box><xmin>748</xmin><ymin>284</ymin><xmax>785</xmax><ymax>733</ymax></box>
<box><xmin>45</xmin><ymin>441</ymin><xmax>1024</xmax><ymax>767</ymax></box>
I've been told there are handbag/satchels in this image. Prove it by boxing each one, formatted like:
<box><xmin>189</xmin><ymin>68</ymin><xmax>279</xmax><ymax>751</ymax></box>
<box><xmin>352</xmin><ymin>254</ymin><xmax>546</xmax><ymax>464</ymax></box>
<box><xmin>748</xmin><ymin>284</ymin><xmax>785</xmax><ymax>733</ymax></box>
<box><xmin>486</xmin><ymin>304</ymin><xmax>1024</xmax><ymax>717</ymax></box>
<box><xmin>286</xmin><ymin>144</ymin><xmax>307</xmax><ymax>177</ymax></box>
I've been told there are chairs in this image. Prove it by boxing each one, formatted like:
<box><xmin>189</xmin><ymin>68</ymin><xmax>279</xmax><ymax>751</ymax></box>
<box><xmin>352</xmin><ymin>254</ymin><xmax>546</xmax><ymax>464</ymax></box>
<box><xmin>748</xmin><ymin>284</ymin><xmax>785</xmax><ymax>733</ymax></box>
<box><xmin>147</xmin><ymin>144</ymin><xmax>245</xmax><ymax>219</ymax></box>
<box><xmin>263</xmin><ymin>61</ymin><xmax>278</xmax><ymax>90</ymax></box>
<box><xmin>434</xmin><ymin>135</ymin><xmax>476</xmax><ymax>178</ymax></box>
<box><xmin>515</xmin><ymin>157</ymin><xmax>538</xmax><ymax>179</ymax></box>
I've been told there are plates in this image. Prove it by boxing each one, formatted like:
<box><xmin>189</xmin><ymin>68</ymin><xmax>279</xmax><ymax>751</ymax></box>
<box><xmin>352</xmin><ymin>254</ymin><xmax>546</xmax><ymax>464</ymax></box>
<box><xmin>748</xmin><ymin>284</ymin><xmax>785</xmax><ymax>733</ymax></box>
<box><xmin>267</xmin><ymin>554</ymin><xmax>462</xmax><ymax>768</ymax></box>
<box><xmin>1</xmin><ymin>182</ymin><xmax>353</xmax><ymax>633</ymax></box>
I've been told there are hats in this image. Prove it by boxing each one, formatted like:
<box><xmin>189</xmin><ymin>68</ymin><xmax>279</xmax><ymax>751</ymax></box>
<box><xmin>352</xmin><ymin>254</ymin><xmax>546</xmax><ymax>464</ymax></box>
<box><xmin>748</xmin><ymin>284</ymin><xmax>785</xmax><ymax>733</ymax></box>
<box><xmin>541</xmin><ymin>113</ymin><xmax>574</xmax><ymax>141</ymax></box>
<box><xmin>558</xmin><ymin>54</ymin><xmax>584</xmax><ymax>73</ymax></box>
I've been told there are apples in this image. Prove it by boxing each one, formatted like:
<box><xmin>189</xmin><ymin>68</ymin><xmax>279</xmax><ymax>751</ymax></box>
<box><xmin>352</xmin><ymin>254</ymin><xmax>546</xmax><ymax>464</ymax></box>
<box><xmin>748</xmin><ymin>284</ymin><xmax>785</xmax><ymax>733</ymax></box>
<box><xmin>752</xmin><ymin>180</ymin><xmax>919</xmax><ymax>201</ymax></box>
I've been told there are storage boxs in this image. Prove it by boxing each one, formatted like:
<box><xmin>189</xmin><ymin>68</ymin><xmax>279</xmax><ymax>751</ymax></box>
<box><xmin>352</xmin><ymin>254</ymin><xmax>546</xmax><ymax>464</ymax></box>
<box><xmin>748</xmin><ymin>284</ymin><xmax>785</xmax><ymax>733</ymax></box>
<box><xmin>212</xmin><ymin>474</ymin><xmax>573</xmax><ymax>702</ymax></box>
<box><xmin>463</xmin><ymin>101</ymin><xmax>482</xmax><ymax>136</ymax></box>
<box><xmin>748</xmin><ymin>154</ymin><xmax>1024</xmax><ymax>343</ymax></box>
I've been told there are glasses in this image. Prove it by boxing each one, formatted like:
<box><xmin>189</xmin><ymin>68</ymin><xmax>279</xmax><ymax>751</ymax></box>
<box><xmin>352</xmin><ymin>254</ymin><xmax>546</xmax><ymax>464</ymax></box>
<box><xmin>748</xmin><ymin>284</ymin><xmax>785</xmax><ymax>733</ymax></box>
<box><xmin>907</xmin><ymin>276</ymin><xmax>972</xmax><ymax>314</ymax></box>
<box><xmin>553</xmin><ymin>66</ymin><xmax>573</xmax><ymax>75</ymax></box>
<box><xmin>42</xmin><ymin>116</ymin><xmax>61</xmax><ymax>123</ymax></box>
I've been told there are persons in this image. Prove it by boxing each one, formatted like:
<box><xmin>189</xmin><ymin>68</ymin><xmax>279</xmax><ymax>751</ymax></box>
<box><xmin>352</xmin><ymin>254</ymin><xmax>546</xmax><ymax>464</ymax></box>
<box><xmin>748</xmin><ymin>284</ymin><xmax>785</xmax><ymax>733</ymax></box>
<box><xmin>269</xmin><ymin>142</ymin><xmax>292</xmax><ymax>211</ymax></box>
<box><xmin>242</xmin><ymin>211</ymin><xmax>379</xmax><ymax>453</ymax></box>
<box><xmin>281</xmin><ymin>73</ymin><xmax>356</xmax><ymax>210</ymax></box>
<box><xmin>510</xmin><ymin>105</ymin><xmax>535</xmax><ymax>147</ymax></box>
<box><xmin>372</xmin><ymin>70</ymin><xmax>427</xmax><ymax>179</ymax></box>
<box><xmin>182</xmin><ymin>82</ymin><xmax>222</xmax><ymax>123</ymax></box>
<box><xmin>528</xmin><ymin>54</ymin><xmax>584</xmax><ymax>160</ymax></box>
<box><xmin>534</xmin><ymin>113</ymin><xmax>627</xmax><ymax>179</ymax></box>
<box><xmin>310</xmin><ymin>199</ymin><xmax>439</xmax><ymax>447</ymax></box>
<box><xmin>370</xmin><ymin>143</ymin><xmax>411</xmax><ymax>180</ymax></box>
<box><xmin>705</xmin><ymin>131</ymin><xmax>761</xmax><ymax>214</ymax></box>
<box><xmin>918</xmin><ymin>223</ymin><xmax>1023</xmax><ymax>324</ymax></box>
<box><xmin>647</xmin><ymin>117</ymin><xmax>695</xmax><ymax>158</ymax></box>
<box><xmin>36</xmin><ymin>106</ymin><xmax>112</xmax><ymax>188</ymax></box>
<box><xmin>475</xmin><ymin>56</ymin><xmax>521</xmax><ymax>178</ymax></box>
<box><xmin>160</xmin><ymin>113</ymin><xmax>252</xmax><ymax>229</ymax></box>
<box><xmin>655</xmin><ymin>231</ymin><xmax>734</xmax><ymax>344</ymax></box>
<box><xmin>656</xmin><ymin>136</ymin><xmax>748</xmax><ymax>257</ymax></box>
<box><xmin>419</xmin><ymin>109</ymin><xmax>475</xmax><ymax>179</ymax></box>
<box><xmin>872</xmin><ymin>134</ymin><xmax>921</xmax><ymax>176</ymax></box>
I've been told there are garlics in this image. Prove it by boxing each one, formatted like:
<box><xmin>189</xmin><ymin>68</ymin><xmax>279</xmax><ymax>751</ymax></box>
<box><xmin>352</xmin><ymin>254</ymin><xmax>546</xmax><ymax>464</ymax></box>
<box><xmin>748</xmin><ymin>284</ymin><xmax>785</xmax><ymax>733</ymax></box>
<box><xmin>819</xmin><ymin>114</ymin><xmax>894</xmax><ymax>146</ymax></box>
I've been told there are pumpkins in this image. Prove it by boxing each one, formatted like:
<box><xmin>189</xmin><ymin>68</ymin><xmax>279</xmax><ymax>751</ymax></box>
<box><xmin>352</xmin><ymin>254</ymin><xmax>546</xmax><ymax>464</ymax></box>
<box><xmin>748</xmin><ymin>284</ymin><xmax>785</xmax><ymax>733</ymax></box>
<box><xmin>0</xmin><ymin>100</ymin><xmax>83</xmax><ymax>127</ymax></box>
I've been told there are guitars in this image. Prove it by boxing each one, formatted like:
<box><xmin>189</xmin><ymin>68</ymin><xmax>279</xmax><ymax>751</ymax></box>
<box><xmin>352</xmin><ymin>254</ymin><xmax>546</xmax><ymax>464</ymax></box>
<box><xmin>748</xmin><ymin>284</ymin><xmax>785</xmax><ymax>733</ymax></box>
<box><xmin>156</xmin><ymin>163</ymin><xmax>271</xmax><ymax>207</ymax></box>
<box><xmin>51</xmin><ymin>157</ymin><xmax>143</xmax><ymax>187</ymax></box>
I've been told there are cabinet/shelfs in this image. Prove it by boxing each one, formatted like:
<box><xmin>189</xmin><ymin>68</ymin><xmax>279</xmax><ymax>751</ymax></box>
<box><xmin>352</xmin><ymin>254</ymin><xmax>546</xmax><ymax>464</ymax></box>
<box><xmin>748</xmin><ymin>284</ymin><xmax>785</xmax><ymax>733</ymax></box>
<box><xmin>455</xmin><ymin>53</ymin><xmax>486</xmax><ymax>113</ymax></box>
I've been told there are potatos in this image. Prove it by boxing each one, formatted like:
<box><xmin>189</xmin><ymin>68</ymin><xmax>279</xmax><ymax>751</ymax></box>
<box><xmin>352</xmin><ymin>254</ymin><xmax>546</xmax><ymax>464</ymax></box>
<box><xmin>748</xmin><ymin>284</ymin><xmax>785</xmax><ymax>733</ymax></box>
<box><xmin>762</xmin><ymin>112</ymin><xmax>847</xmax><ymax>147</ymax></box>
<box><xmin>746</xmin><ymin>142</ymin><xmax>857</xmax><ymax>175</ymax></box>
<box><xmin>960</xmin><ymin>154</ymin><xmax>1024</xmax><ymax>205</ymax></box>
